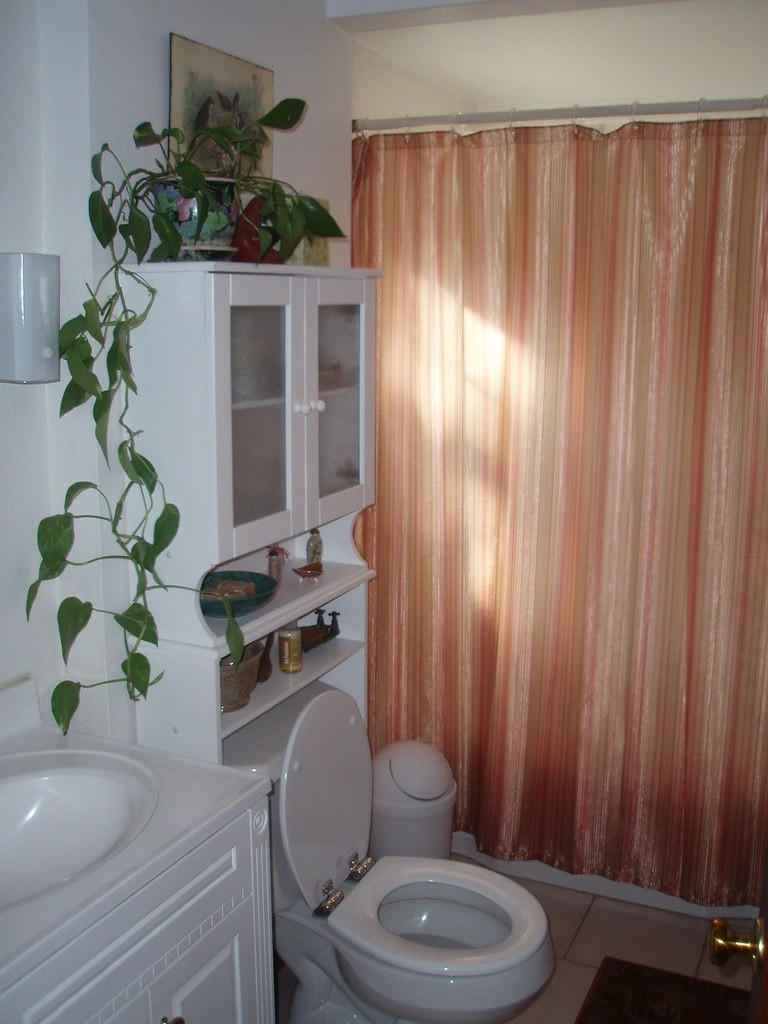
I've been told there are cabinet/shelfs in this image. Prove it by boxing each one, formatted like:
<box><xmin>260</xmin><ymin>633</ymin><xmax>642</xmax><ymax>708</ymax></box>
<box><xmin>113</xmin><ymin>262</ymin><xmax>384</xmax><ymax>766</ymax></box>
<box><xmin>0</xmin><ymin>797</ymin><xmax>275</xmax><ymax>1024</ymax></box>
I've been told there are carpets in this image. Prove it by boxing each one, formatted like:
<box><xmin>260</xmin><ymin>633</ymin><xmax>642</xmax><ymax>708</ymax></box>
<box><xmin>574</xmin><ymin>957</ymin><xmax>749</xmax><ymax>1024</ymax></box>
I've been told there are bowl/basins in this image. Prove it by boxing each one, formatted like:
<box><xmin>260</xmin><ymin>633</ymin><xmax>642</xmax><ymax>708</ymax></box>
<box><xmin>200</xmin><ymin>570</ymin><xmax>280</xmax><ymax>619</ymax></box>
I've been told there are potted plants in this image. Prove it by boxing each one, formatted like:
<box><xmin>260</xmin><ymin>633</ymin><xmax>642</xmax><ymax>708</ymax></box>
<box><xmin>26</xmin><ymin>99</ymin><xmax>347</xmax><ymax>739</ymax></box>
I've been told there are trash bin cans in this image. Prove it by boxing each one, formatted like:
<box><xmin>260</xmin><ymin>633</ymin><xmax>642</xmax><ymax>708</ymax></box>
<box><xmin>368</xmin><ymin>740</ymin><xmax>457</xmax><ymax>862</ymax></box>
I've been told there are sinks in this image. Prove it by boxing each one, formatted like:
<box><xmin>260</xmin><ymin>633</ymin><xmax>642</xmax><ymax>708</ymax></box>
<box><xmin>1</xmin><ymin>725</ymin><xmax>163</xmax><ymax>918</ymax></box>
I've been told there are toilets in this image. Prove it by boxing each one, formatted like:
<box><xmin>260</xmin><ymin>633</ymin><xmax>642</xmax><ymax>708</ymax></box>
<box><xmin>222</xmin><ymin>680</ymin><xmax>557</xmax><ymax>1024</ymax></box>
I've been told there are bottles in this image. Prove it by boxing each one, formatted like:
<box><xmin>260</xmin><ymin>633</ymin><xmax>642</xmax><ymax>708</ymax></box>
<box><xmin>278</xmin><ymin>620</ymin><xmax>303</xmax><ymax>674</ymax></box>
<box><xmin>268</xmin><ymin>552</ymin><xmax>279</xmax><ymax>581</ymax></box>
<box><xmin>306</xmin><ymin>528</ymin><xmax>323</xmax><ymax>564</ymax></box>
